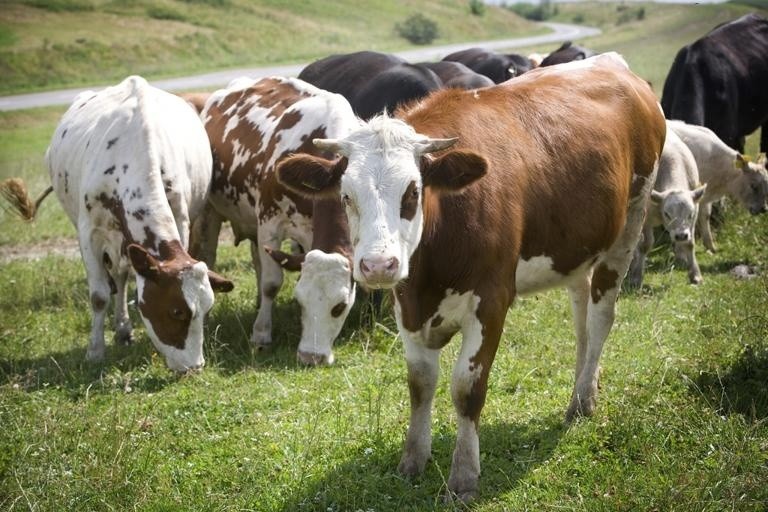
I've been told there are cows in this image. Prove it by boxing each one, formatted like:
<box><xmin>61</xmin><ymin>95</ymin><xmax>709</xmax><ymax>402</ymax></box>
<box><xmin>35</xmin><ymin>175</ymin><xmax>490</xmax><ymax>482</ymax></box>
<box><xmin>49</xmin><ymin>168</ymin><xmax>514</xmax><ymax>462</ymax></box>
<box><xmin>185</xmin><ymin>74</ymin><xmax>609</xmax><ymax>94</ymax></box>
<box><xmin>0</xmin><ymin>12</ymin><xmax>768</xmax><ymax>374</ymax></box>
<box><xmin>276</xmin><ymin>52</ymin><xmax>666</xmax><ymax>507</ymax></box>
